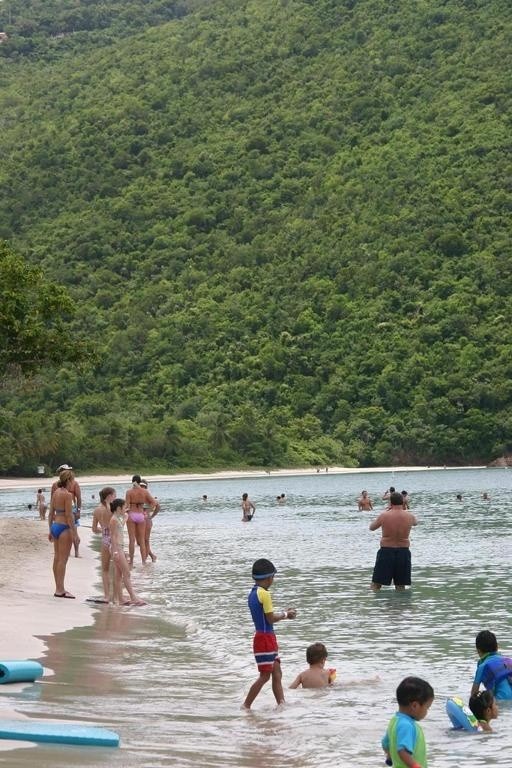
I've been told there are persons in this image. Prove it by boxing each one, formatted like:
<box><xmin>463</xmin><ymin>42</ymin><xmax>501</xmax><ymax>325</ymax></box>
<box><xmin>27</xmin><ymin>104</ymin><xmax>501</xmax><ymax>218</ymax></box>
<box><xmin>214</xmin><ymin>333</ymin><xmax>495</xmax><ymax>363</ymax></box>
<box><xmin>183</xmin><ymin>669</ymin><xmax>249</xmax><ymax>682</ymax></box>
<box><xmin>35</xmin><ymin>489</ymin><xmax>48</xmax><ymax>520</ymax></box>
<box><xmin>290</xmin><ymin>642</ymin><xmax>336</xmax><ymax>689</ymax></box>
<box><xmin>466</xmin><ymin>690</ymin><xmax>498</xmax><ymax>731</ymax></box>
<box><xmin>49</xmin><ymin>462</ymin><xmax>160</xmax><ymax>605</ymax></box>
<box><xmin>456</xmin><ymin>494</ymin><xmax>463</xmax><ymax>501</ymax></box>
<box><xmin>281</xmin><ymin>493</ymin><xmax>287</xmax><ymax>501</ymax></box>
<box><xmin>239</xmin><ymin>558</ymin><xmax>298</xmax><ymax>712</ymax></box>
<box><xmin>470</xmin><ymin>630</ymin><xmax>512</xmax><ymax>700</ymax></box>
<box><xmin>358</xmin><ymin>490</ymin><xmax>373</xmax><ymax>511</ymax></box>
<box><xmin>401</xmin><ymin>490</ymin><xmax>409</xmax><ymax>510</ymax></box>
<box><xmin>382</xmin><ymin>677</ymin><xmax>435</xmax><ymax>767</ymax></box>
<box><xmin>241</xmin><ymin>492</ymin><xmax>256</xmax><ymax>522</ymax></box>
<box><xmin>481</xmin><ymin>492</ymin><xmax>490</xmax><ymax>501</ymax></box>
<box><xmin>277</xmin><ymin>496</ymin><xmax>285</xmax><ymax>506</ymax></box>
<box><xmin>367</xmin><ymin>492</ymin><xmax>419</xmax><ymax>592</ymax></box>
<box><xmin>28</xmin><ymin>504</ymin><xmax>33</xmax><ymax>510</ymax></box>
<box><xmin>203</xmin><ymin>495</ymin><xmax>209</xmax><ymax>502</ymax></box>
<box><xmin>382</xmin><ymin>486</ymin><xmax>396</xmax><ymax>508</ymax></box>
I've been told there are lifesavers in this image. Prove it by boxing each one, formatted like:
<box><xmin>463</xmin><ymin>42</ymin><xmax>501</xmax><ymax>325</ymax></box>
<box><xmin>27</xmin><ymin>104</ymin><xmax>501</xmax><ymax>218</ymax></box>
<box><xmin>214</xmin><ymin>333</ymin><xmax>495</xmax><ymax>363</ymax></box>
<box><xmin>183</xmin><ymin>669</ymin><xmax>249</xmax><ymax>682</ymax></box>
<box><xmin>447</xmin><ymin>697</ymin><xmax>483</xmax><ymax>732</ymax></box>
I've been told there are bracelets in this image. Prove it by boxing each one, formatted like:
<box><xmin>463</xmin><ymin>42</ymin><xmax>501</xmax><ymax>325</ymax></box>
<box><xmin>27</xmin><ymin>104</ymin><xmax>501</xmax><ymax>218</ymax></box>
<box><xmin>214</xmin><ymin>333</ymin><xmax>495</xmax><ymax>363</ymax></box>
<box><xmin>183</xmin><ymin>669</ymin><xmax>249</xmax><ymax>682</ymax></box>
<box><xmin>282</xmin><ymin>611</ymin><xmax>290</xmax><ymax>619</ymax></box>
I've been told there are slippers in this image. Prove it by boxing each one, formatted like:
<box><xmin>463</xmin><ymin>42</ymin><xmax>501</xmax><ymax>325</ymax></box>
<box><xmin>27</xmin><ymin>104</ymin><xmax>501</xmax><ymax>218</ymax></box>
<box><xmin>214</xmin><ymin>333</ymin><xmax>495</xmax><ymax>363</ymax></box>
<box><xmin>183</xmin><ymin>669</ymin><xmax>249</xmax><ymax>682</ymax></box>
<box><xmin>54</xmin><ymin>592</ymin><xmax>76</xmax><ymax>599</ymax></box>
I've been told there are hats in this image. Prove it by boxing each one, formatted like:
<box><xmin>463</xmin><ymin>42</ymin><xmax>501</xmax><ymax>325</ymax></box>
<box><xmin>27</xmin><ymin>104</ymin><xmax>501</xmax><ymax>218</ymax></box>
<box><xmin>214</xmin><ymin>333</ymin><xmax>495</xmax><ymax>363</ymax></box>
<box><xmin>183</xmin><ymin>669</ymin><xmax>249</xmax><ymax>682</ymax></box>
<box><xmin>56</xmin><ymin>464</ymin><xmax>72</xmax><ymax>471</ymax></box>
